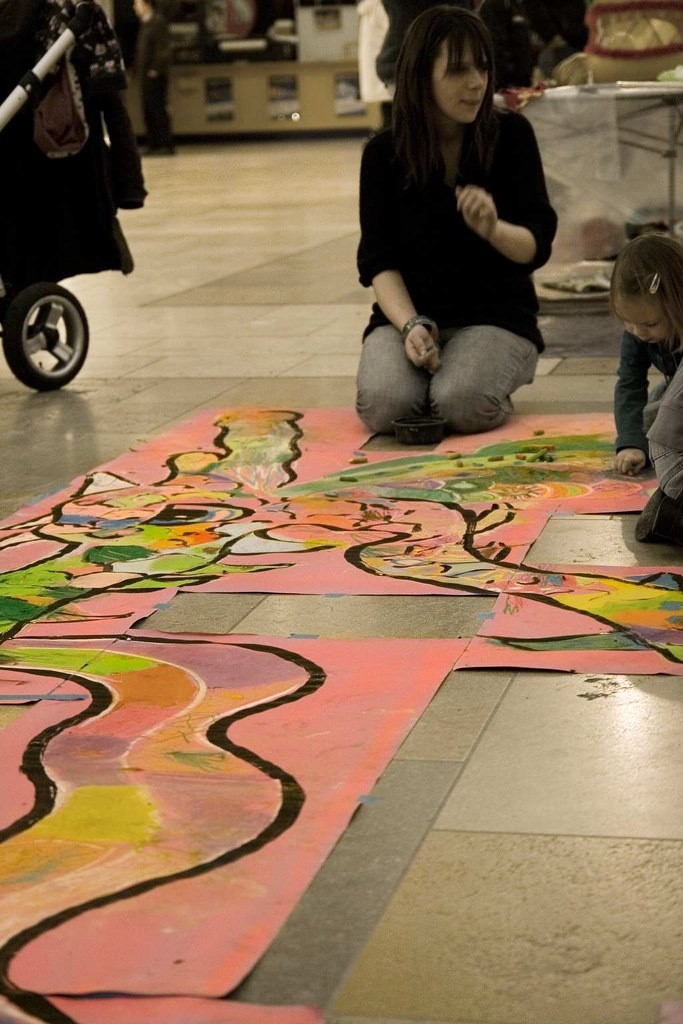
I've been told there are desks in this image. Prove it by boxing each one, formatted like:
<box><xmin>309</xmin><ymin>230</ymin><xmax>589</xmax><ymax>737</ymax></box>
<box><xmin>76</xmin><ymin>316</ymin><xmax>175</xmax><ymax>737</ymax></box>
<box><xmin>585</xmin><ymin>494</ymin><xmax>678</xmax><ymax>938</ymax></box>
<box><xmin>495</xmin><ymin>77</ymin><xmax>683</xmax><ymax>241</ymax></box>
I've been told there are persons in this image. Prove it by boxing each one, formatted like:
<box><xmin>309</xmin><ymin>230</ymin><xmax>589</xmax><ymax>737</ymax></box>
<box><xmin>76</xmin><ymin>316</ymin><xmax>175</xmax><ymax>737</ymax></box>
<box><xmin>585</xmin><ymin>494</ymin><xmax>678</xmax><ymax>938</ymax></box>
<box><xmin>59</xmin><ymin>1</ymin><xmax>174</xmax><ymax>153</ymax></box>
<box><xmin>610</xmin><ymin>234</ymin><xmax>683</xmax><ymax>543</ymax></box>
<box><xmin>357</xmin><ymin>7</ymin><xmax>557</xmax><ymax>431</ymax></box>
<box><xmin>376</xmin><ymin>0</ymin><xmax>588</xmax><ymax>96</ymax></box>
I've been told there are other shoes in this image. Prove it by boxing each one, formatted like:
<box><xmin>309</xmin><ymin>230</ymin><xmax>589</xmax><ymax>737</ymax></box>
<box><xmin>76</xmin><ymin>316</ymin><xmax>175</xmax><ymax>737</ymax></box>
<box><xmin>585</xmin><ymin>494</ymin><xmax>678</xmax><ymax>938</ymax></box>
<box><xmin>145</xmin><ymin>147</ymin><xmax>175</xmax><ymax>156</ymax></box>
<box><xmin>635</xmin><ymin>486</ymin><xmax>665</xmax><ymax>540</ymax></box>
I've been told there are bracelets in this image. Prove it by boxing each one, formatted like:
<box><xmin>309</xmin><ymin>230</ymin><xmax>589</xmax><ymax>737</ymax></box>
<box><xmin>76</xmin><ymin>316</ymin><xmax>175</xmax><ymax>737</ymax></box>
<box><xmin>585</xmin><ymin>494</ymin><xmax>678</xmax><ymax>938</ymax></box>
<box><xmin>402</xmin><ymin>316</ymin><xmax>439</xmax><ymax>344</ymax></box>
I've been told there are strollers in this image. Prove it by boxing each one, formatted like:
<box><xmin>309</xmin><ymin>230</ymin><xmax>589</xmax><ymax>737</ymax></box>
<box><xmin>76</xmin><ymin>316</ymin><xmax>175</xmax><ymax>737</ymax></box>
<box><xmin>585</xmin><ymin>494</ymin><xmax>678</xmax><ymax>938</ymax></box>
<box><xmin>0</xmin><ymin>0</ymin><xmax>147</xmax><ymax>393</ymax></box>
